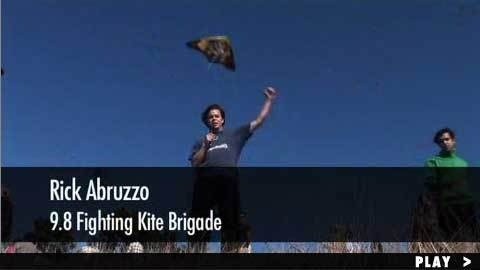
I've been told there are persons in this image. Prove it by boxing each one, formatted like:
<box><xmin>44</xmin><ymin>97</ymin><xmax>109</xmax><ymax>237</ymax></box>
<box><xmin>236</xmin><ymin>211</ymin><xmax>252</xmax><ymax>252</ymax></box>
<box><xmin>188</xmin><ymin>87</ymin><xmax>278</xmax><ymax>253</ymax></box>
<box><xmin>424</xmin><ymin>126</ymin><xmax>471</xmax><ymax>239</ymax></box>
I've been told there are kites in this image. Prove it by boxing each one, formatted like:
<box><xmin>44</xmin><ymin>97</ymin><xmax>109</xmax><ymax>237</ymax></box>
<box><xmin>184</xmin><ymin>33</ymin><xmax>235</xmax><ymax>74</ymax></box>
